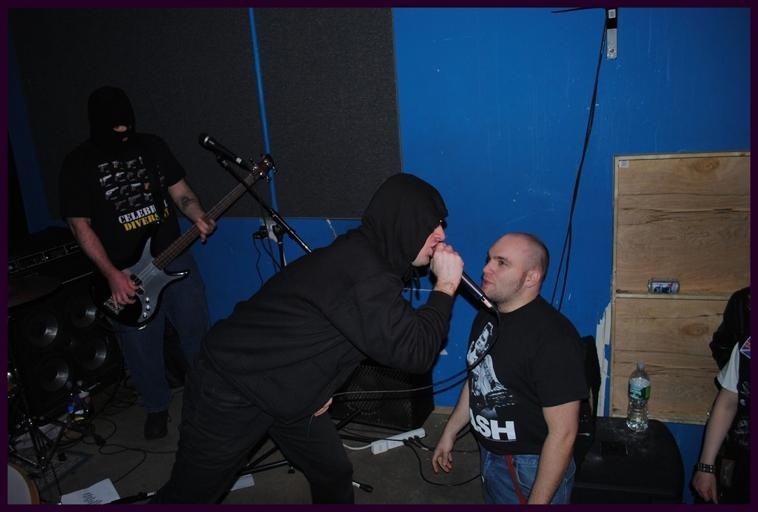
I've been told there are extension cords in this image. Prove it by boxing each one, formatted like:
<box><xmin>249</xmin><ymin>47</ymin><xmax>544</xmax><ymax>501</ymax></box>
<box><xmin>371</xmin><ymin>427</ymin><xmax>426</xmax><ymax>455</ymax></box>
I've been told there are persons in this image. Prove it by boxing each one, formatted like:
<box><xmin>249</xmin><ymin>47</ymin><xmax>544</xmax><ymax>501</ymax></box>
<box><xmin>147</xmin><ymin>171</ymin><xmax>467</xmax><ymax>503</ymax></box>
<box><xmin>431</xmin><ymin>231</ymin><xmax>592</xmax><ymax>504</ymax></box>
<box><xmin>59</xmin><ymin>83</ymin><xmax>219</xmax><ymax>440</ymax></box>
<box><xmin>690</xmin><ymin>334</ymin><xmax>751</xmax><ymax>504</ymax></box>
<box><xmin>707</xmin><ymin>286</ymin><xmax>751</xmax><ymax>370</ymax></box>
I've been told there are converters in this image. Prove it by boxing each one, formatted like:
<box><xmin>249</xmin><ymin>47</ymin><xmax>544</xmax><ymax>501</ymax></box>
<box><xmin>252</xmin><ymin>227</ymin><xmax>266</xmax><ymax>240</ymax></box>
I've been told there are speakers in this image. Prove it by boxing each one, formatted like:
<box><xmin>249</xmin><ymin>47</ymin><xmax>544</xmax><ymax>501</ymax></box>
<box><xmin>7</xmin><ymin>276</ymin><xmax>127</xmax><ymax>427</ymax></box>
<box><xmin>327</xmin><ymin>356</ymin><xmax>434</xmax><ymax>432</ymax></box>
<box><xmin>570</xmin><ymin>415</ymin><xmax>685</xmax><ymax>504</ymax></box>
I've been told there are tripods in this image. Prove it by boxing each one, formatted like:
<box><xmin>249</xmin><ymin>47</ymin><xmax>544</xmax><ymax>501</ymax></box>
<box><xmin>7</xmin><ymin>351</ymin><xmax>96</xmax><ymax>473</ymax></box>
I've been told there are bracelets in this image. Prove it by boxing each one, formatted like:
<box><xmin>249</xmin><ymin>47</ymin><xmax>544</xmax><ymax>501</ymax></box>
<box><xmin>696</xmin><ymin>461</ymin><xmax>716</xmax><ymax>474</ymax></box>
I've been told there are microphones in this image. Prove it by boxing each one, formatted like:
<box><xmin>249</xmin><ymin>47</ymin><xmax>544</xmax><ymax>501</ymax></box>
<box><xmin>197</xmin><ymin>132</ymin><xmax>272</xmax><ymax>185</ymax></box>
<box><xmin>460</xmin><ymin>271</ymin><xmax>496</xmax><ymax>311</ymax></box>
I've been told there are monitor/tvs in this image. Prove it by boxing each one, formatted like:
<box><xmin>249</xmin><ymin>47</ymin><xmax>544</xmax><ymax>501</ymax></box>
<box><xmin>217</xmin><ymin>160</ymin><xmax>375</xmax><ymax>493</ymax></box>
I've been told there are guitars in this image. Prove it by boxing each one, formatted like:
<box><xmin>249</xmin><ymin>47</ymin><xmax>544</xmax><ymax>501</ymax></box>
<box><xmin>95</xmin><ymin>151</ymin><xmax>277</xmax><ymax>326</ymax></box>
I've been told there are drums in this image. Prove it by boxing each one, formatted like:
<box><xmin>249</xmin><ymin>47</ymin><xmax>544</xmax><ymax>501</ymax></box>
<box><xmin>7</xmin><ymin>460</ymin><xmax>40</xmax><ymax>505</ymax></box>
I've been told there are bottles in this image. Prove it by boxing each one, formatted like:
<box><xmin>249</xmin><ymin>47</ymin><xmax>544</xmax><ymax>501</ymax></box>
<box><xmin>626</xmin><ymin>362</ymin><xmax>651</xmax><ymax>432</ymax></box>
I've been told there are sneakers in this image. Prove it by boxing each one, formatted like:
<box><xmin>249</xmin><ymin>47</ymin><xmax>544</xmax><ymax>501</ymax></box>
<box><xmin>145</xmin><ymin>409</ymin><xmax>171</xmax><ymax>438</ymax></box>
